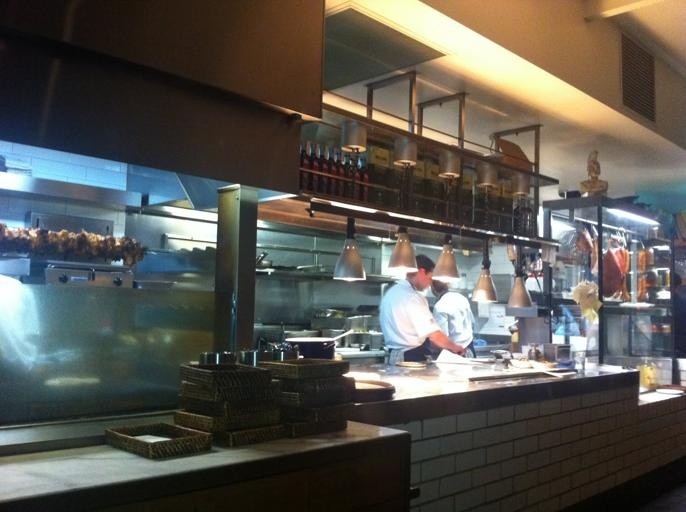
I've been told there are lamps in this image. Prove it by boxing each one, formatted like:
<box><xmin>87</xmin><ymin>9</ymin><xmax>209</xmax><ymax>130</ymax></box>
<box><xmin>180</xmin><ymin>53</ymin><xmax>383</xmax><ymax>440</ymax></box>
<box><xmin>505</xmin><ymin>244</ymin><xmax>533</xmax><ymax>310</ymax></box>
<box><xmin>331</xmin><ymin>218</ymin><xmax>366</xmax><ymax>283</ymax></box>
<box><xmin>470</xmin><ymin>239</ymin><xmax>499</xmax><ymax>305</ymax></box>
<box><xmin>430</xmin><ymin>234</ymin><xmax>459</xmax><ymax>282</ymax></box>
<box><xmin>388</xmin><ymin>223</ymin><xmax>421</xmax><ymax>275</ymax></box>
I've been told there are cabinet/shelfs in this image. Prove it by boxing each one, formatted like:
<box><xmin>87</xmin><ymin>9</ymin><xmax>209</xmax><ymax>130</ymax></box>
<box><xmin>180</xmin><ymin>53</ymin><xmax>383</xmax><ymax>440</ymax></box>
<box><xmin>541</xmin><ymin>197</ymin><xmax>686</xmax><ymax>394</ymax></box>
<box><xmin>295</xmin><ymin>86</ymin><xmax>563</xmax><ymax>253</ymax></box>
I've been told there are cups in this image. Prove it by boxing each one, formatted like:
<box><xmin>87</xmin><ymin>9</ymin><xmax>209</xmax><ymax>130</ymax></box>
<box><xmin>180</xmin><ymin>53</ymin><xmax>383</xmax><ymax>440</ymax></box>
<box><xmin>569</xmin><ymin>342</ymin><xmax>587</xmax><ymax>371</ymax></box>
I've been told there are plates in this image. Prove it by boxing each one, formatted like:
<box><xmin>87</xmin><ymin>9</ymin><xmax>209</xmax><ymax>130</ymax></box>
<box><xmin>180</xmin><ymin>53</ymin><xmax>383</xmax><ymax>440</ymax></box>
<box><xmin>352</xmin><ymin>380</ymin><xmax>396</xmax><ymax>400</ymax></box>
<box><xmin>395</xmin><ymin>361</ymin><xmax>427</xmax><ymax>367</ymax></box>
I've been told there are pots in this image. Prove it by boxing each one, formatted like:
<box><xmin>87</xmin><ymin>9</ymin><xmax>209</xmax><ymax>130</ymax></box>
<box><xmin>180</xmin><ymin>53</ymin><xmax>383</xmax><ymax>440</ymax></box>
<box><xmin>201</xmin><ymin>305</ymin><xmax>386</xmax><ymax>366</ymax></box>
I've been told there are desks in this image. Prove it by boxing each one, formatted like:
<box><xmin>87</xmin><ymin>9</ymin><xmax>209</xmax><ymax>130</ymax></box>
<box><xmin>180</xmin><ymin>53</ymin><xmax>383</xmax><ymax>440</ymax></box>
<box><xmin>0</xmin><ymin>419</ymin><xmax>413</xmax><ymax>510</ymax></box>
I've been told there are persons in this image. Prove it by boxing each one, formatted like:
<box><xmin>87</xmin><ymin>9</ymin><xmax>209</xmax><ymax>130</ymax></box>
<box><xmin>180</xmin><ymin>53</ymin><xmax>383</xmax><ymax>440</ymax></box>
<box><xmin>380</xmin><ymin>254</ymin><xmax>468</xmax><ymax>365</ymax></box>
<box><xmin>431</xmin><ymin>280</ymin><xmax>480</xmax><ymax>359</ymax></box>
<box><xmin>1</xmin><ymin>274</ymin><xmax>40</xmax><ymax>379</ymax></box>
<box><xmin>675</xmin><ymin>271</ymin><xmax>686</xmax><ymax>359</ymax></box>
<box><xmin>645</xmin><ymin>272</ymin><xmax>675</xmax><ymax>357</ymax></box>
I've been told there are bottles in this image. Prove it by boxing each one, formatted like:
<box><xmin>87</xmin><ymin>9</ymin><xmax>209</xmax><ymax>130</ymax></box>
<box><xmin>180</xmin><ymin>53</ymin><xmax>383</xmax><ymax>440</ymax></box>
<box><xmin>461</xmin><ymin>272</ymin><xmax>467</xmax><ymax>289</ymax></box>
<box><xmin>298</xmin><ymin>144</ymin><xmax>370</xmax><ymax>204</ymax></box>
<box><xmin>530</xmin><ymin>343</ymin><xmax>543</xmax><ymax>361</ymax></box>
